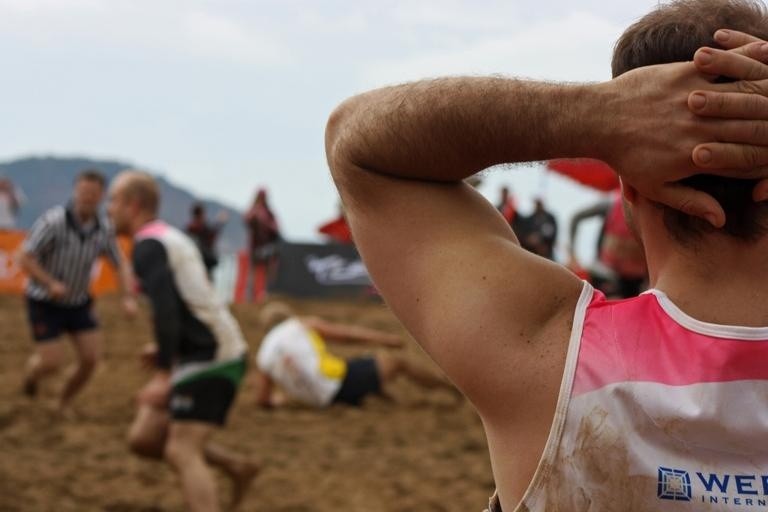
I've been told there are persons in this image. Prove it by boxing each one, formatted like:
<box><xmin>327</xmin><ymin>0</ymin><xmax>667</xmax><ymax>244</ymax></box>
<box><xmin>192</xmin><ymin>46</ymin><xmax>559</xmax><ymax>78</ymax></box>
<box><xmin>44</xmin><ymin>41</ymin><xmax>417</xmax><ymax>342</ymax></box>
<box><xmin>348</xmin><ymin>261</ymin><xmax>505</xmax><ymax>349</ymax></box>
<box><xmin>494</xmin><ymin>187</ymin><xmax>557</xmax><ymax>260</ymax></box>
<box><xmin>246</xmin><ymin>190</ymin><xmax>283</xmax><ymax>305</ymax></box>
<box><xmin>187</xmin><ymin>201</ymin><xmax>232</xmax><ymax>282</ymax></box>
<box><xmin>324</xmin><ymin>0</ymin><xmax>768</xmax><ymax>512</ymax></box>
<box><xmin>1</xmin><ymin>175</ymin><xmax>25</xmax><ymax>230</ymax></box>
<box><xmin>253</xmin><ymin>301</ymin><xmax>462</xmax><ymax>408</ymax></box>
<box><xmin>107</xmin><ymin>169</ymin><xmax>261</xmax><ymax>511</ymax></box>
<box><xmin>14</xmin><ymin>167</ymin><xmax>141</xmax><ymax>410</ymax></box>
